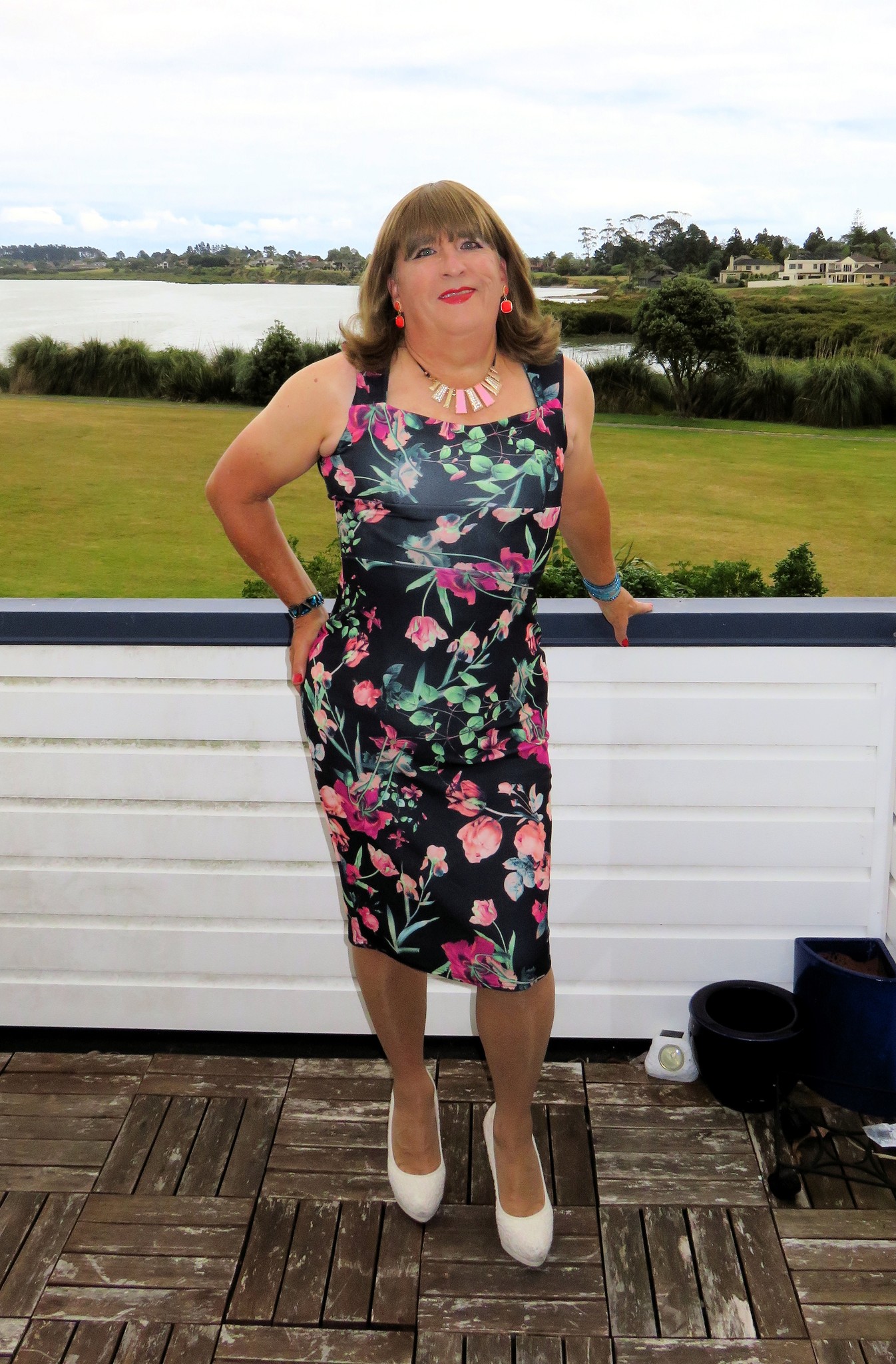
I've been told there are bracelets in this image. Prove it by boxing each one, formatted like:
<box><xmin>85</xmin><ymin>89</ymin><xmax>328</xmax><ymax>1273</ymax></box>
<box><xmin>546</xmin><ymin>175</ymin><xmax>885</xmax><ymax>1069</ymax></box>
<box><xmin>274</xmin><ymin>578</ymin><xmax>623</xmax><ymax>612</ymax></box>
<box><xmin>287</xmin><ymin>590</ymin><xmax>324</xmax><ymax>619</ymax></box>
<box><xmin>582</xmin><ymin>572</ymin><xmax>622</xmax><ymax>601</ymax></box>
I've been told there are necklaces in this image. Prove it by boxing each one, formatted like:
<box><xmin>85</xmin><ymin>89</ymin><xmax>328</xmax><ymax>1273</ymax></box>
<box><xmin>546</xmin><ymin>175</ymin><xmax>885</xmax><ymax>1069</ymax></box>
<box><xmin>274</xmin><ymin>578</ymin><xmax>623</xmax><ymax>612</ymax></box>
<box><xmin>416</xmin><ymin>353</ymin><xmax>503</xmax><ymax>414</ymax></box>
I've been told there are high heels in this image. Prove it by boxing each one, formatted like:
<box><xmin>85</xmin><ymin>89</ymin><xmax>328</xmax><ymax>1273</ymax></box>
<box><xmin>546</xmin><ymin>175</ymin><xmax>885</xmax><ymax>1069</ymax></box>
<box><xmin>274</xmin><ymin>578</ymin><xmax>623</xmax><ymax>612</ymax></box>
<box><xmin>387</xmin><ymin>1071</ymin><xmax>446</xmax><ymax>1222</ymax></box>
<box><xmin>482</xmin><ymin>1103</ymin><xmax>555</xmax><ymax>1268</ymax></box>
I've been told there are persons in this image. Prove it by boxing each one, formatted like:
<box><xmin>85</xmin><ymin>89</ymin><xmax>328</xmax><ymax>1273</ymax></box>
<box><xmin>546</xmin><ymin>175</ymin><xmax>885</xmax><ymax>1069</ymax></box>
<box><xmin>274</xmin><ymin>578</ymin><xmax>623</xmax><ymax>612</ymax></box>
<box><xmin>203</xmin><ymin>177</ymin><xmax>652</xmax><ymax>1268</ymax></box>
